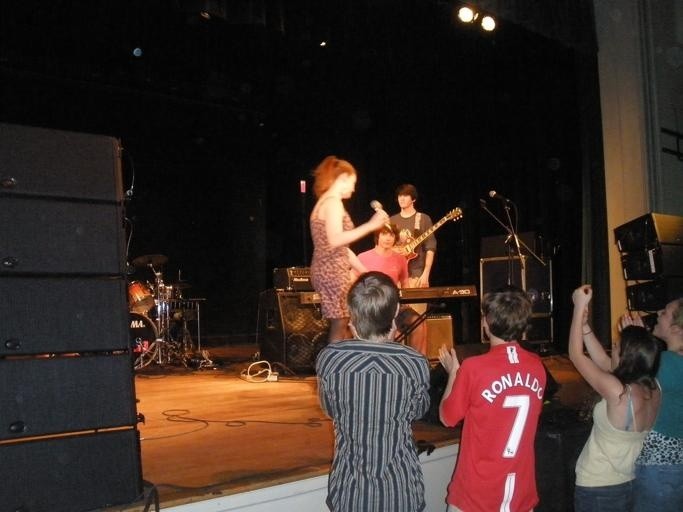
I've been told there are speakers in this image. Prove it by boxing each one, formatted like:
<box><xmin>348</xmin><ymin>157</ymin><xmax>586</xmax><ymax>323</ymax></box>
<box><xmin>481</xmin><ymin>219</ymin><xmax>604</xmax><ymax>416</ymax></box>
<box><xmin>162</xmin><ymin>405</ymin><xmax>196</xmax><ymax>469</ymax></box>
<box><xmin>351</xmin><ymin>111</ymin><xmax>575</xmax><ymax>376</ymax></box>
<box><xmin>614</xmin><ymin>212</ymin><xmax>683</xmax><ymax>311</ymax></box>
<box><xmin>257</xmin><ymin>289</ymin><xmax>332</xmax><ymax>377</ymax></box>
<box><xmin>0</xmin><ymin>124</ymin><xmax>145</xmax><ymax>511</ymax></box>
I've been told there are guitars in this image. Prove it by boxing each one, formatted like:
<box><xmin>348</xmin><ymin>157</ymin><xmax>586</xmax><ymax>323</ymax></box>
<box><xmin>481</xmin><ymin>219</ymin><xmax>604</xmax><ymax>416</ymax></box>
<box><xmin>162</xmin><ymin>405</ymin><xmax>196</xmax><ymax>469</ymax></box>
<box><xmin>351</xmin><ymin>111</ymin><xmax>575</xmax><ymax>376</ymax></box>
<box><xmin>391</xmin><ymin>208</ymin><xmax>463</xmax><ymax>263</ymax></box>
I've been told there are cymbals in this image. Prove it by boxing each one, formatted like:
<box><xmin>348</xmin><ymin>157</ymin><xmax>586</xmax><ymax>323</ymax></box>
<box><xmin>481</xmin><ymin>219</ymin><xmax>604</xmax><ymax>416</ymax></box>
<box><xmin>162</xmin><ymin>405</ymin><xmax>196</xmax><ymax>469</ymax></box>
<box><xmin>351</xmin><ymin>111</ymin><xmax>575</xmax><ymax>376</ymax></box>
<box><xmin>174</xmin><ymin>283</ymin><xmax>191</xmax><ymax>289</ymax></box>
<box><xmin>132</xmin><ymin>254</ymin><xmax>169</xmax><ymax>268</ymax></box>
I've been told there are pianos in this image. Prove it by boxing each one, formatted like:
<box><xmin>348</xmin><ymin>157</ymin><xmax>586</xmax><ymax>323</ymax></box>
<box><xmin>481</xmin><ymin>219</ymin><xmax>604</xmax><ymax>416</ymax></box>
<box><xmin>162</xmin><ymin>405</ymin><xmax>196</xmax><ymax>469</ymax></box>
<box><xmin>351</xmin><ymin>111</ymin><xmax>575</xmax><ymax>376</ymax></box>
<box><xmin>297</xmin><ymin>284</ymin><xmax>477</xmax><ymax>304</ymax></box>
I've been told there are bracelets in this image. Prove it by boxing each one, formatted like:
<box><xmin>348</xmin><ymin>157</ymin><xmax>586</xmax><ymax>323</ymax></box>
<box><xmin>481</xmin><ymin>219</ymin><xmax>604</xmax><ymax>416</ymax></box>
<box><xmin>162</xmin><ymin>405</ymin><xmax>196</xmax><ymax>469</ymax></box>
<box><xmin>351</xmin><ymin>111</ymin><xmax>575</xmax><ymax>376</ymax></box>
<box><xmin>583</xmin><ymin>330</ymin><xmax>593</xmax><ymax>335</ymax></box>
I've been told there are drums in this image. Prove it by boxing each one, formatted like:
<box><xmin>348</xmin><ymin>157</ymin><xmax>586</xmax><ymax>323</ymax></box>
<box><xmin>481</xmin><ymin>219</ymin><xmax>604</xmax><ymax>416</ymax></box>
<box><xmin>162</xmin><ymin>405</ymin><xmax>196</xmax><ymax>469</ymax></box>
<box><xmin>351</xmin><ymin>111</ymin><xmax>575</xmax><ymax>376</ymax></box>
<box><xmin>127</xmin><ymin>280</ymin><xmax>155</xmax><ymax>313</ymax></box>
<box><xmin>150</xmin><ymin>317</ymin><xmax>166</xmax><ymax>337</ymax></box>
<box><xmin>129</xmin><ymin>311</ymin><xmax>159</xmax><ymax>370</ymax></box>
<box><xmin>151</xmin><ymin>286</ymin><xmax>182</xmax><ymax>299</ymax></box>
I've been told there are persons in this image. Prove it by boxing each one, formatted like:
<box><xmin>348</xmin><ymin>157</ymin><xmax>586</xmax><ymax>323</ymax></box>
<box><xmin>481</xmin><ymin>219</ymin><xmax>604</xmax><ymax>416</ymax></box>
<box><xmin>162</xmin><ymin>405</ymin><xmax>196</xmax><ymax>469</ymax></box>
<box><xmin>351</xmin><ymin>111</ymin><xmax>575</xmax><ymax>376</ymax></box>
<box><xmin>310</xmin><ymin>157</ymin><xmax>437</xmax><ymax>357</ymax></box>
<box><xmin>568</xmin><ymin>285</ymin><xmax>663</xmax><ymax>512</ymax></box>
<box><xmin>618</xmin><ymin>297</ymin><xmax>683</xmax><ymax>511</ymax></box>
<box><xmin>315</xmin><ymin>271</ymin><xmax>431</xmax><ymax>512</ymax></box>
<box><xmin>438</xmin><ymin>285</ymin><xmax>547</xmax><ymax>511</ymax></box>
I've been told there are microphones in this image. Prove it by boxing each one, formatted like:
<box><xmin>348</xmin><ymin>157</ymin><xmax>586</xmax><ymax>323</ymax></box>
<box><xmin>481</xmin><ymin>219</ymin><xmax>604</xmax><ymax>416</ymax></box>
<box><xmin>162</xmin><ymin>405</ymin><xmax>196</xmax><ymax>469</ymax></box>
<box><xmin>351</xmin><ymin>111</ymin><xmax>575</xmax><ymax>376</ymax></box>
<box><xmin>489</xmin><ymin>190</ymin><xmax>509</xmax><ymax>203</ymax></box>
<box><xmin>370</xmin><ymin>200</ymin><xmax>390</xmax><ymax>230</ymax></box>
